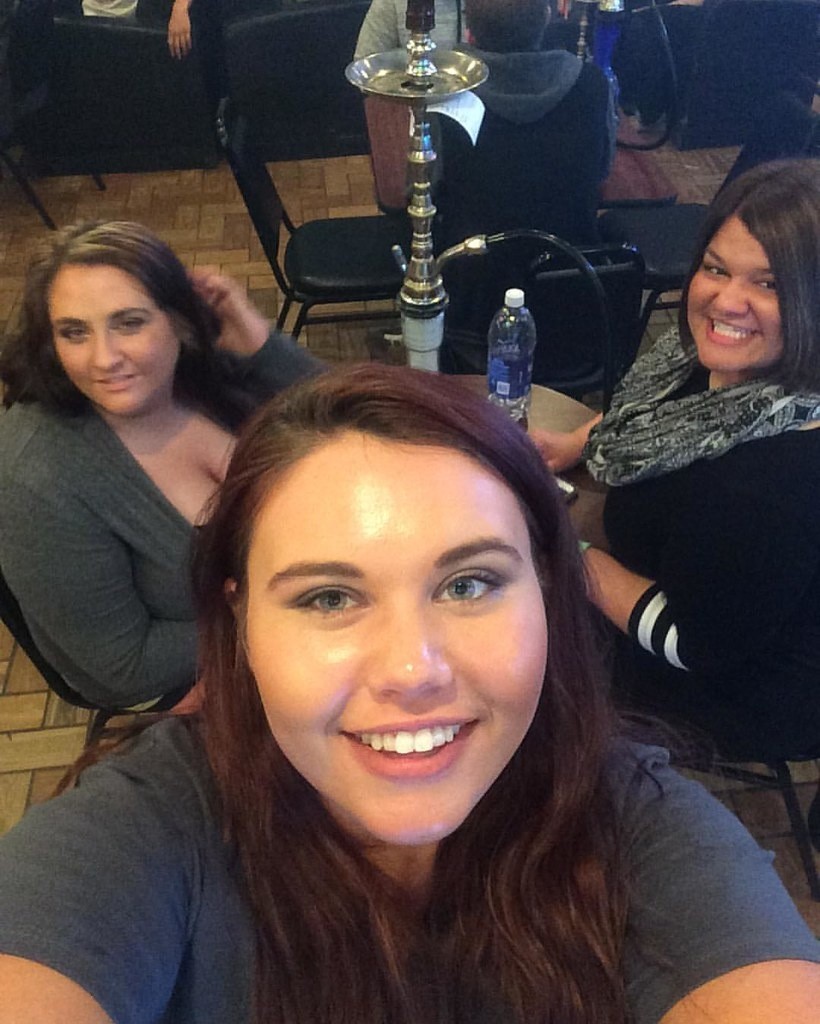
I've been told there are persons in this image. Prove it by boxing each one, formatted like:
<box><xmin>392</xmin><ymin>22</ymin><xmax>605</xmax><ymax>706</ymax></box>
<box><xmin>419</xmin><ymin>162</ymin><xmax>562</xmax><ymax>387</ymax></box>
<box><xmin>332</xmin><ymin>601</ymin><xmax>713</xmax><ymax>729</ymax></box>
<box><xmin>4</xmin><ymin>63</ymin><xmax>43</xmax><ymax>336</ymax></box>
<box><xmin>0</xmin><ymin>216</ymin><xmax>353</xmax><ymax>716</ymax></box>
<box><xmin>528</xmin><ymin>156</ymin><xmax>820</xmax><ymax>771</ymax></box>
<box><xmin>0</xmin><ymin>351</ymin><xmax>819</xmax><ymax>1024</ymax></box>
<box><xmin>0</xmin><ymin>0</ymin><xmax>820</xmax><ymax>192</ymax></box>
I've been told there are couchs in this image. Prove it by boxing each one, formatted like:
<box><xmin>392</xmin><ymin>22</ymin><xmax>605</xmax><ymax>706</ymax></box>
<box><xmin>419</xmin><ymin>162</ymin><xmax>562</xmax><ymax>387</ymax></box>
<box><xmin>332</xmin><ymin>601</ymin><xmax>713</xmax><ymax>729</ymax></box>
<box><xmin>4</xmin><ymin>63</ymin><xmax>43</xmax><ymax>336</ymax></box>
<box><xmin>213</xmin><ymin>0</ymin><xmax>369</xmax><ymax>163</ymax></box>
<box><xmin>612</xmin><ymin>0</ymin><xmax>819</xmax><ymax>153</ymax></box>
<box><xmin>11</xmin><ymin>0</ymin><xmax>219</xmax><ymax>179</ymax></box>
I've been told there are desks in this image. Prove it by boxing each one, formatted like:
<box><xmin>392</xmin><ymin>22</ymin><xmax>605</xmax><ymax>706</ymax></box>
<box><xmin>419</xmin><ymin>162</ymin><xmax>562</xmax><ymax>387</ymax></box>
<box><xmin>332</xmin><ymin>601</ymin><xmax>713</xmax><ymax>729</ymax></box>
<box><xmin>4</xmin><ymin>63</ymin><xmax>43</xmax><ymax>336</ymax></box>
<box><xmin>363</xmin><ymin>94</ymin><xmax>681</xmax><ymax>217</ymax></box>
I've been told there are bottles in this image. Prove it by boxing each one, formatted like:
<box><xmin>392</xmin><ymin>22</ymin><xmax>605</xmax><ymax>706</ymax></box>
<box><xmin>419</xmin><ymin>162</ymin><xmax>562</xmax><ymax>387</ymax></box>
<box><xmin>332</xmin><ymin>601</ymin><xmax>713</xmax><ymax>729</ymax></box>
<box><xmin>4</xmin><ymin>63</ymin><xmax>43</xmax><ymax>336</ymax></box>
<box><xmin>487</xmin><ymin>289</ymin><xmax>536</xmax><ymax>434</ymax></box>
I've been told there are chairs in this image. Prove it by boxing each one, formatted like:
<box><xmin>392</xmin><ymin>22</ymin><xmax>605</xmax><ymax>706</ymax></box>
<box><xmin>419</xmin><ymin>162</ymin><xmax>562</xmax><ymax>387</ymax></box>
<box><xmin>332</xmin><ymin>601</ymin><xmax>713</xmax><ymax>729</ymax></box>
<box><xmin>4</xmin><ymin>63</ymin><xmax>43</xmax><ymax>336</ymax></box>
<box><xmin>599</xmin><ymin>71</ymin><xmax>820</xmax><ymax>375</ymax></box>
<box><xmin>446</xmin><ymin>240</ymin><xmax>647</xmax><ymax>414</ymax></box>
<box><xmin>0</xmin><ymin>0</ymin><xmax>106</xmax><ymax>230</ymax></box>
<box><xmin>609</xmin><ymin>686</ymin><xmax>820</xmax><ymax>903</ymax></box>
<box><xmin>215</xmin><ymin>94</ymin><xmax>413</xmax><ymax>341</ymax></box>
<box><xmin>0</xmin><ymin>570</ymin><xmax>194</xmax><ymax>747</ymax></box>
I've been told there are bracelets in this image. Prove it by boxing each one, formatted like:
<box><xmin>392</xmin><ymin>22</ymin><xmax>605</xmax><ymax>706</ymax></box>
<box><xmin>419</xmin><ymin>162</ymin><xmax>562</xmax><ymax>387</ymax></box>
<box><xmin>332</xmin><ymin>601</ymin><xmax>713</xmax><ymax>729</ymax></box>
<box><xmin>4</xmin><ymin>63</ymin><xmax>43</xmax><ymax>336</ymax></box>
<box><xmin>576</xmin><ymin>540</ymin><xmax>592</xmax><ymax>553</ymax></box>
